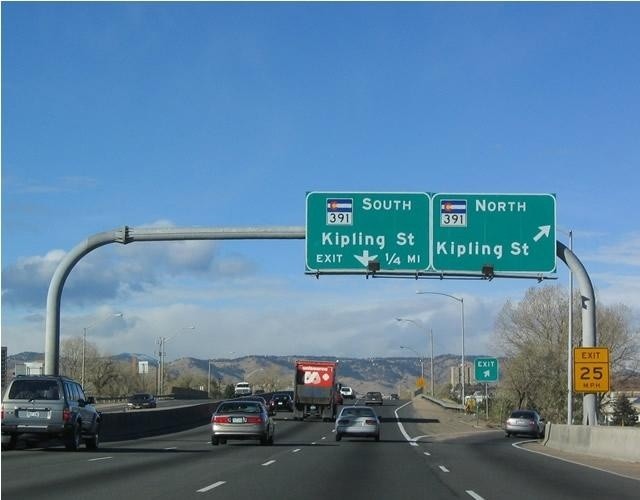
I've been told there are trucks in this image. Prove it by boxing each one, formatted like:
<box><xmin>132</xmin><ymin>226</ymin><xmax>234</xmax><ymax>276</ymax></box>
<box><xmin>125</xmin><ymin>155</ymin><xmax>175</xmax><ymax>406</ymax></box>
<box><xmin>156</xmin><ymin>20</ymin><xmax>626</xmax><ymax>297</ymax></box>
<box><xmin>292</xmin><ymin>360</ymin><xmax>337</xmax><ymax>422</ymax></box>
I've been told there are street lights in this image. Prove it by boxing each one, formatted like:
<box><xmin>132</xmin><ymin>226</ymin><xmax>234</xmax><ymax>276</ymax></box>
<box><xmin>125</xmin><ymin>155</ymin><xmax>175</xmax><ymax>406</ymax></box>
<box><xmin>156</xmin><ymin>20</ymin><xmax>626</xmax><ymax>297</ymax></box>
<box><xmin>395</xmin><ymin>317</ymin><xmax>434</xmax><ymax>397</ymax></box>
<box><xmin>244</xmin><ymin>367</ymin><xmax>264</xmax><ymax>384</ymax></box>
<box><xmin>206</xmin><ymin>352</ymin><xmax>234</xmax><ymax>396</ymax></box>
<box><xmin>160</xmin><ymin>325</ymin><xmax>195</xmax><ymax>394</ymax></box>
<box><xmin>398</xmin><ymin>344</ymin><xmax>425</xmax><ymax>379</ymax></box>
<box><xmin>81</xmin><ymin>313</ymin><xmax>123</xmax><ymax>392</ymax></box>
<box><xmin>416</xmin><ymin>288</ymin><xmax>465</xmax><ymax>410</ymax></box>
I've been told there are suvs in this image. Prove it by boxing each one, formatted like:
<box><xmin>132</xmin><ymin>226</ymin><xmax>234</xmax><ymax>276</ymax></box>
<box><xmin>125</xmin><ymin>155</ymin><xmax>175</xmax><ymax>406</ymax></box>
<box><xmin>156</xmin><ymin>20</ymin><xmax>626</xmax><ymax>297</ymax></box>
<box><xmin>365</xmin><ymin>392</ymin><xmax>384</xmax><ymax>406</ymax></box>
<box><xmin>340</xmin><ymin>387</ymin><xmax>355</xmax><ymax>399</ymax></box>
<box><xmin>235</xmin><ymin>382</ymin><xmax>251</xmax><ymax>397</ymax></box>
<box><xmin>1</xmin><ymin>375</ymin><xmax>101</xmax><ymax>451</ymax></box>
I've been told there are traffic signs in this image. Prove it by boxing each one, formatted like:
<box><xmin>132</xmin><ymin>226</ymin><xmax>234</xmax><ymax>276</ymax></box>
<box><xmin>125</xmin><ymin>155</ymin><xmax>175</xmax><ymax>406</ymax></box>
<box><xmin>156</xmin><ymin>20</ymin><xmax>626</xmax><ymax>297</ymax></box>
<box><xmin>304</xmin><ymin>191</ymin><xmax>431</xmax><ymax>274</ymax></box>
<box><xmin>431</xmin><ymin>193</ymin><xmax>556</xmax><ymax>275</ymax></box>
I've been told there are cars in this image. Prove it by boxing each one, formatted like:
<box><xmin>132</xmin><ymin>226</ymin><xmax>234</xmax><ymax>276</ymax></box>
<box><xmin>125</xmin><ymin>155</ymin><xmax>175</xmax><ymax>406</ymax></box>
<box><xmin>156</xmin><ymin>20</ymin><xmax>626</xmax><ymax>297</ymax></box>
<box><xmin>211</xmin><ymin>400</ymin><xmax>277</xmax><ymax>447</ymax></box>
<box><xmin>239</xmin><ymin>394</ymin><xmax>293</xmax><ymax>414</ymax></box>
<box><xmin>335</xmin><ymin>406</ymin><xmax>382</xmax><ymax>443</ymax></box>
<box><xmin>335</xmin><ymin>391</ymin><xmax>343</xmax><ymax>404</ymax></box>
<box><xmin>390</xmin><ymin>393</ymin><xmax>400</xmax><ymax>400</ymax></box>
<box><xmin>506</xmin><ymin>410</ymin><xmax>545</xmax><ymax>440</ymax></box>
<box><xmin>126</xmin><ymin>393</ymin><xmax>155</xmax><ymax>410</ymax></box>
